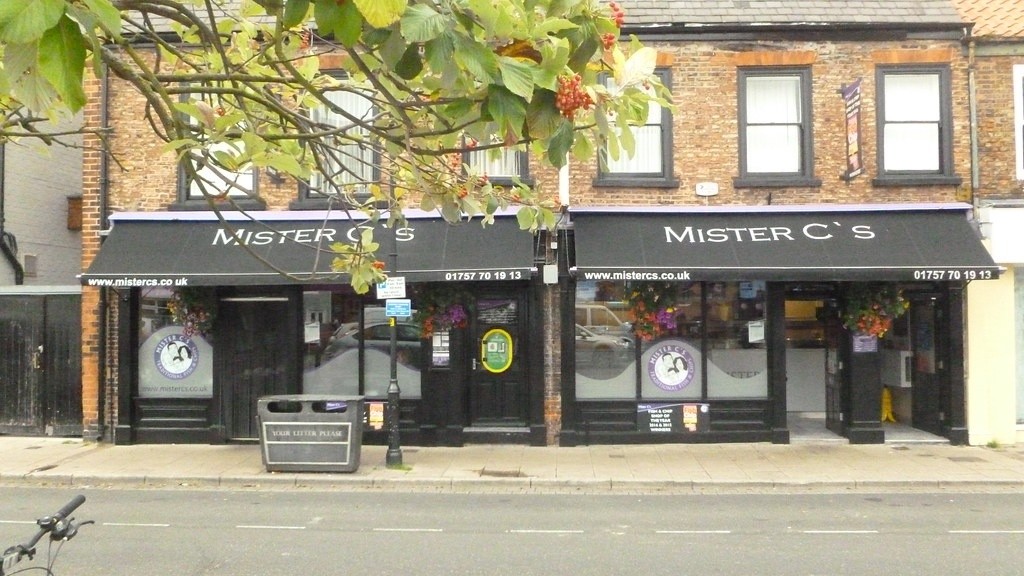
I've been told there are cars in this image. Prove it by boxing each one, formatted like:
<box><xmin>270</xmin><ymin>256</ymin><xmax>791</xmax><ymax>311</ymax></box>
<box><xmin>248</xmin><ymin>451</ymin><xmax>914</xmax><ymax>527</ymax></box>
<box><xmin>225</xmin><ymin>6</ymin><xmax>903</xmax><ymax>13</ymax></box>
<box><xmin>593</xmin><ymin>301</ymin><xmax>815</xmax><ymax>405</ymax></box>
<box><xmin>311</xmin><ymin>297</ymin><xmax>637</xmax><ymax>380</ymax></box>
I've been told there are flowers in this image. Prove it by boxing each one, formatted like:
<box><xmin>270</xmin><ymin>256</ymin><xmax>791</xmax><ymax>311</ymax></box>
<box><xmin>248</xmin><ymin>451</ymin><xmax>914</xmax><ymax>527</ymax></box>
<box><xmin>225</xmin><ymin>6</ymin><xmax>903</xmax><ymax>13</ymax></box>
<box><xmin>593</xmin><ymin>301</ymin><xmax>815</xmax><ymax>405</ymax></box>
<box><xmin>167</xmin><ymin>287</ymin><xmax>220</xmax><ymax>339</ymax></box>
<box><xmin>619</xmin><ymin>281</ymin><xmax>682</xmax><ymax>343</ymax></box>
<box><xmin>836</xmin><ymin>282</ymin><xmax>911</xmax><ymax>338</ymax></box>
<box><xmin>410</xmin><ymin>284</ymin><xmax>479</xmax><ymax>340</ymax></box>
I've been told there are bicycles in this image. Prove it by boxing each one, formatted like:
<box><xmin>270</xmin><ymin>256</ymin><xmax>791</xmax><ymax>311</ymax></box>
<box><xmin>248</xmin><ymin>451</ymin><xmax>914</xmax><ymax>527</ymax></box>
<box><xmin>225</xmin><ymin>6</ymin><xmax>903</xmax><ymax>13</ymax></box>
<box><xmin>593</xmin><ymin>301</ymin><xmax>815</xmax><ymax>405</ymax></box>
<box><xmin>0</xmin><ymin>494</ymin><xmax>95</xmax><ymax>576</ymax></box>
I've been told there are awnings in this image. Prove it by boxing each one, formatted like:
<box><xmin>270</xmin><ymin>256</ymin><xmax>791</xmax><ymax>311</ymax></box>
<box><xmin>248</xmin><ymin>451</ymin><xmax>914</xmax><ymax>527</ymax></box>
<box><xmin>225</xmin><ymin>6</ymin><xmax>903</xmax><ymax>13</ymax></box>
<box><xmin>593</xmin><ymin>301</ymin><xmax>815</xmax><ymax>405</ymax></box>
<box><xmin>76</xmin><ymin>216</ymin><xmax>538</xmax><ymax>287</ymax></box>
<box><xmin>571</xmin><ymin>209</ymin><xmax>1009</xmax><ymax>281</ymax></box>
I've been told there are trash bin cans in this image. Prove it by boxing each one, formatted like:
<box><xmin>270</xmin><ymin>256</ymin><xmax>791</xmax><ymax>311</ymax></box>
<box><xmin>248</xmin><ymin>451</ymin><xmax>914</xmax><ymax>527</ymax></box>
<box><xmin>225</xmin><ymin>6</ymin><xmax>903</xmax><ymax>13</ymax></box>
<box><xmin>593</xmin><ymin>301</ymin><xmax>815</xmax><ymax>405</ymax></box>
<box><xmin>256</xmin><ymin>393</ymin><xmax>364</xmax><ymax>474</ymax></box>
